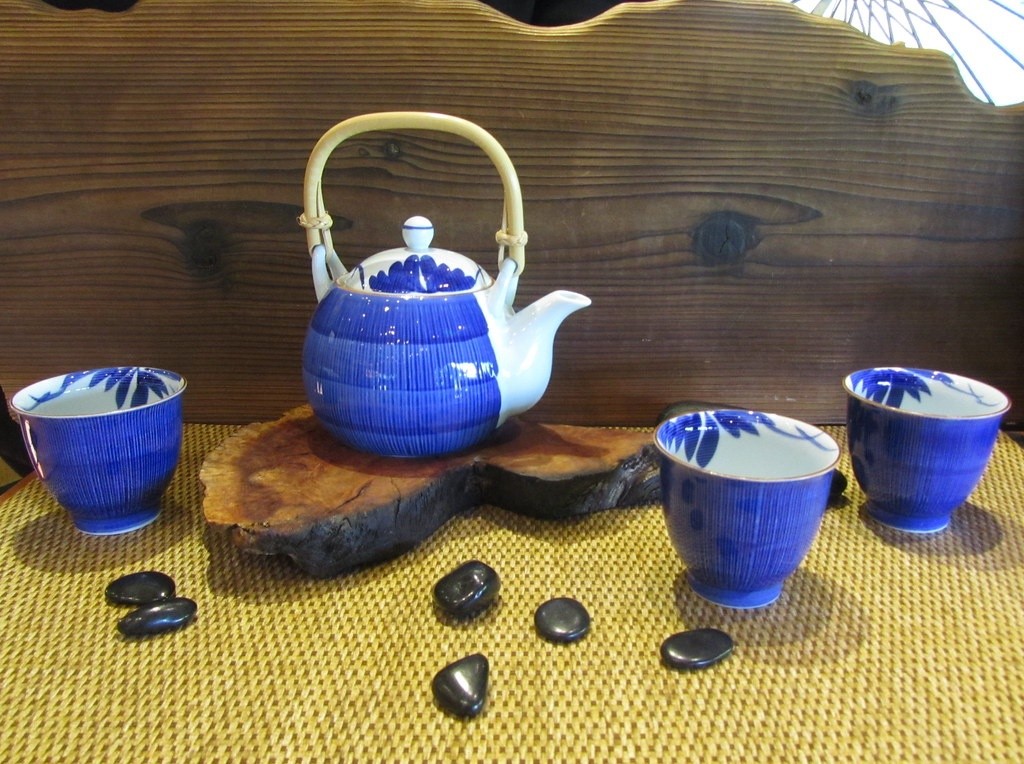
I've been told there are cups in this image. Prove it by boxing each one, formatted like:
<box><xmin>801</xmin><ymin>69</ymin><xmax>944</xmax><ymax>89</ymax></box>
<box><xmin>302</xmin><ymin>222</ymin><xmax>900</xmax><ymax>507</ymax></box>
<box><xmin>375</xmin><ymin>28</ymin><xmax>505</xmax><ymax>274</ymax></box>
<box><xmin>841</xmin><ymin>366</ymin><xmax>1012</xmax><ymax>534</ymax></box>
<box><xmin>9</xmin><ymin>367</ymin><xmax>189</xmax><ymax>535</ymax></box>
<box><xmin>654</xmin><ymin>407</ymin><xmax>842</xmax><ymax>610</ymax></box>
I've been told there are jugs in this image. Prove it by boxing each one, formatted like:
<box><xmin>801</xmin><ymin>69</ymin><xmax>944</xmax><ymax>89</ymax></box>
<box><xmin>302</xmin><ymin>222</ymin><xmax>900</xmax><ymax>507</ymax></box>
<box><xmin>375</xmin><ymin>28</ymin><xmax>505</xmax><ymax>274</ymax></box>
<box><xmin>294</xmin><ymin>109</ymin><xmax>591</xmax><ymax>461</ymax></box>
